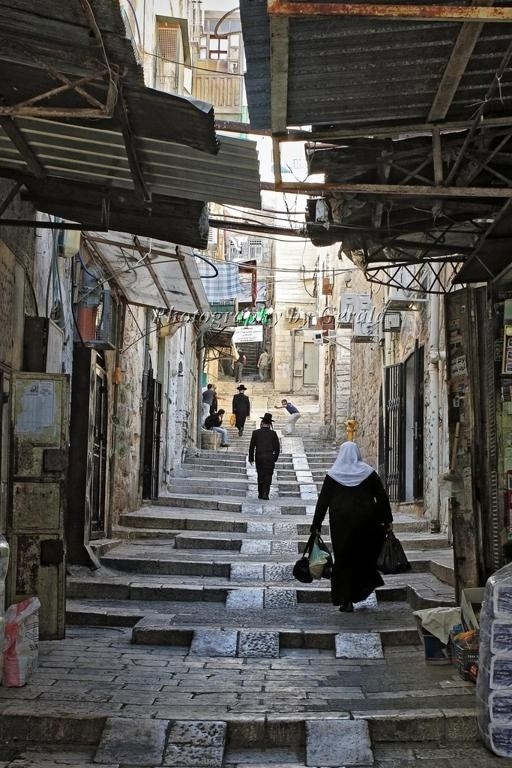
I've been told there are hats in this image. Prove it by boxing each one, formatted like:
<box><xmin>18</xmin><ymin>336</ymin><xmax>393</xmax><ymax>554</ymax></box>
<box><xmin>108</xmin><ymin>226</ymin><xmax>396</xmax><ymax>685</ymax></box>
<box><xmin>237</xmin><ymin>385</ymin><xmax>247</xmax><ymax>390</ymax></box>
<box><xmin>261</xmin><ymin>413</ymin><xmax>275</xmax><ymax>421</ymax></box>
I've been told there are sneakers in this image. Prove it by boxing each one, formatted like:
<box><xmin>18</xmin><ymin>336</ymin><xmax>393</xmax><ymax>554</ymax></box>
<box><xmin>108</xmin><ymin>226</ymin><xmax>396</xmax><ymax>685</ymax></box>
<box><xmin>220</xmin><ymin>443</ymin><xmax>230</xmax><ymax>447</ymax></box>
<box><xmin>339</xmin><ymin>602</ymin><xmax>354</xmax><ymax>613</ymax></box>
<box><xmin>238</xmin><ymin>427</ymin><xmax>243</xmax><ymax>436</ymax></box>
<box><xmin>258</xmin><ymin>492</ymin><xmax>269</xmax><ymax>500</ymax></box>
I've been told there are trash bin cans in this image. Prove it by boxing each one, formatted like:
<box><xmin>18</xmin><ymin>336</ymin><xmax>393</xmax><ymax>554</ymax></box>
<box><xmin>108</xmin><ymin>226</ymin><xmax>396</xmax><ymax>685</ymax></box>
<box><xmin>201</xmin><ymin>431</ymin><xmax>217</xmax><ymax>450</ymax></box>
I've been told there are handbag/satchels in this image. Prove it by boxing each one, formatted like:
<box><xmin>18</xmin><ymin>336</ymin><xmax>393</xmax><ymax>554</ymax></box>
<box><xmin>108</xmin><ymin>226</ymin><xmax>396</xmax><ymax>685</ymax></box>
<box><xmin>377</xmin><ymin>537</ymin><xmax>412</xmax><ymax>574</ymax></box>
<box><xmin>292</xmin><ymin>541</ymin><xmax>334</xmax><ymax>583</ymax></box>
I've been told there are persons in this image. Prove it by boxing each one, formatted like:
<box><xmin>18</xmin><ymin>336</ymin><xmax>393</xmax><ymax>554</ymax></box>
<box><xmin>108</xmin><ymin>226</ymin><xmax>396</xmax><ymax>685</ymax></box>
<box><xmin>256</xmin><ymin>348</ymin><xmax>273</xmax><ymax>382</ymax></box>
<box><xmin>274</xmin><ymin>399</ymin><xmax>301</xmax><ymax>434</ymax></box>
<box><xmin>309</xmin><ymin>441</ymin><xmax>394</xmax><ymax>611</ymax></box>
<box><xmin>232</xmin><ymin>385</ymin><xmax>250</xmax><ymax>436</ymax></box>
<box><xmin>209</xmin><ymin>392</ymin><xmax>217</xmax><ymax>415</ymax></box>
<box><xmin>200</xmin><ymin>384</ymin><xmax>217</xmax><ymax>425</ymax></box>
<box><xmin>233</xmin><ymin>349</ymin><xmax>247</xmax><ymax>382</ymax></box>
<box><xmin>238</xmin><ymin>277</ymin><xmax>252</xmax><ymax>302</ymax></box>
<box><xmin>249</xmin><ymin>413</ymin><xmax>280</xmax><ymax>500</ymax></box>
<box><xmin>256</xmin><ymin>347</ymin><xmax>264</xmax><ymax>363</ymax></box>
<box><xmin>205</xmin><ymin>409</ymin><xmax>230</xmax><ymax>447</ymax></box>
